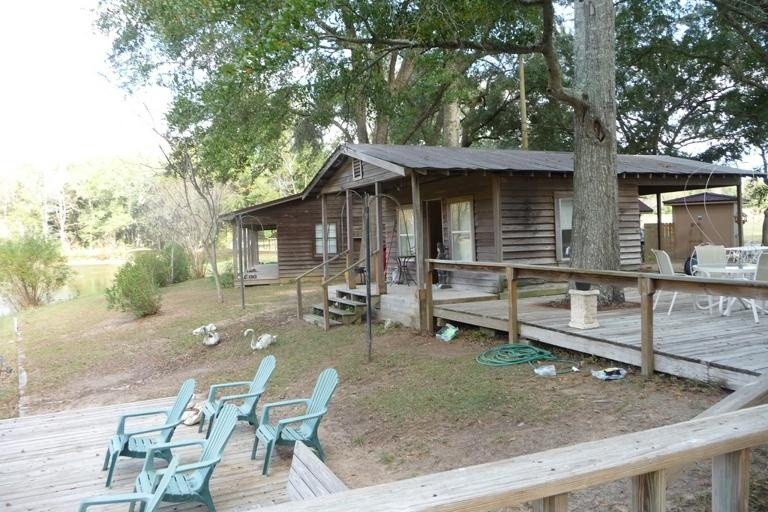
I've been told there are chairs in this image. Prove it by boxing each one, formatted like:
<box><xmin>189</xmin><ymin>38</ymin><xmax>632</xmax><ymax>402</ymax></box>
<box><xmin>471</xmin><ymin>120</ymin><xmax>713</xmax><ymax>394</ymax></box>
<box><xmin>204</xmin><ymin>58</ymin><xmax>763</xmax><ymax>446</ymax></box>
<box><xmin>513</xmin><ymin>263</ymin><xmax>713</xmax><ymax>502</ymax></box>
<box><xmin>80</xmin><ymin>453</ymin><xmax>181</xmax><ymax>510</ymax></box>
<box><xmin>199</xmin><ymin>356</ymin><xmax>276</xmax><ymax>440</ymax></box>
<box><xmin>249</xmin><ymin>367</ymin><xmax>339</xmax><ymax>476</ymax></box>
<box><xmin>648</xmin><ymin>243</ymin><xmax>767</xmax><ymax>323</ymax></box>
<box><xmin>101</xmin><ymin>378</ymin><xmax>195</xmax><ymax>487</ymax></box>
<box><xmin>128</xmin><ymin>405</ymin><xmax>237</xmax><ymax>512</ymax></box>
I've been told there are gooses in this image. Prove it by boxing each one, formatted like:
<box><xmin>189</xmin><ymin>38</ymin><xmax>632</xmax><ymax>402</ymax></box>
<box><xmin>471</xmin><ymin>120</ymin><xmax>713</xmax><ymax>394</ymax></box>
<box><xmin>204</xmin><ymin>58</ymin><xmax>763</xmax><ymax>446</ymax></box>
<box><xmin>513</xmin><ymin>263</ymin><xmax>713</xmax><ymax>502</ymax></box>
<box><xmin>192</xmin><ymin>323</ymin><xmax>217</xmax><ymax>335</ymax></box>
<box><xmin>201</xmin><ymin>327</ymin><xmax>219</xmax><ymax>347</ymax></box>
<box><xmin>243</xmin><ymin>327</ymin><xmax>279</xmax><ymax>351</ymax></box>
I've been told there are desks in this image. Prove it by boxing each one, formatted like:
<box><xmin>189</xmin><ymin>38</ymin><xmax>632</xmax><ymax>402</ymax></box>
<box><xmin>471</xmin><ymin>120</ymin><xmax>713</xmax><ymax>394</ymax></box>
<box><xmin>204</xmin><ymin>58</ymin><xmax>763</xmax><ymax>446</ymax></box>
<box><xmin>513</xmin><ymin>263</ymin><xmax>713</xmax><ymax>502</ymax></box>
<box><xmin>389</xmin><ymin>255</ymin><xmax>416</xmax><ymax>287</ymax></box>
